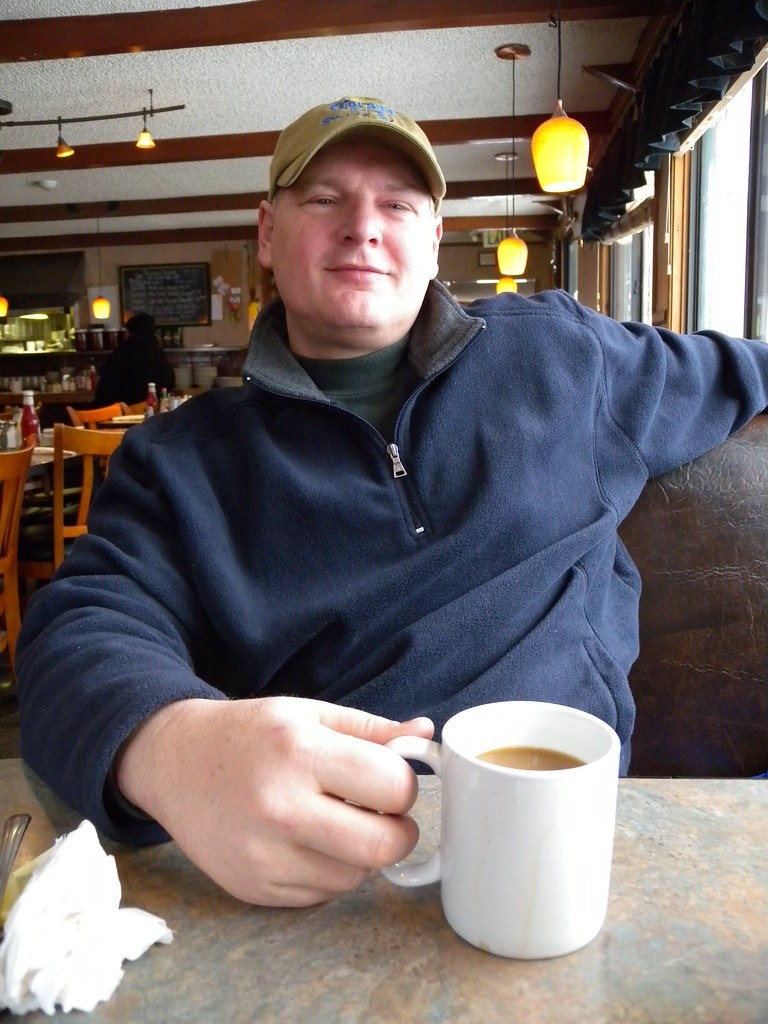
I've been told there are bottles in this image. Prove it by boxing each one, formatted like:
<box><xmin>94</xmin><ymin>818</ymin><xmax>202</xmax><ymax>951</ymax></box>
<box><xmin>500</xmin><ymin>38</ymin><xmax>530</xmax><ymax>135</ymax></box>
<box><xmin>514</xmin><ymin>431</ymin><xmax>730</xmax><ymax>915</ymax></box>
<box><xmin>0</xmin><ymin>420</ymin><xmax>6</xmax><ymax>451</ymax></box>
<box><xmin>21</xmin><ymin>390</ymin><xmax>39</xmax><ymax>448</ymax></box>
<box><xmin>90</xmin><ymin>365</ymin><xmax>96</xmax><ymax>390</ymax></box>
<box><xmin>105</xmin><ymin>328</ymin><xmax>121</xmax><ymax>349</ymax></box>
<box><xmin>62</xmin><ymin>375</ymin><xmax>70</xmax><ymax>391</ymax></box>
<box><xmin>89</xmin><ymin>328</ymin><xmax>104</xmax><ymax>350</ymax></box>
<box><xmin>70</xmin><ymin>378</ymin><xmax>76</xmax><ymax>390</ymax></box>
<box><xmin>170</xmin><ymin>394</ymin><xmax>178</xmax><ymax>409</ymax></box>
<box><xmin>147</xmin><ymin>407</ymin><xmax>153</xmax><ymax>418</ymax></box>
<box><xmin>24</xmin><ymin>376</ymin><xmax>47</xmax><ymax>392</ymax></box>
<box><xmin>145</xmin><ymin>383</ymin><xmax>159</xmax><ymax>416</ymax></box>
<box><xmin>6</xmin><ymin>421</ymin><xmax>19</xmax><ymax>450</ymax></box>
<box><xmin>160</xmin><ymin>388</ymin><xmax>169</xmax><ymax>413</ymax></box>
<box><xmin>86</xmin><ymin>375</ymin><xmax>92</xmax><ymax>390</ymax></box>
<box><xmin>82</xmin><ymin>370</ymin><xmax>87</xmax><ymax>389</ymax></box>
<box><xmin>75</xmin><ymin>329</ymin><xmax>89</xmax><ymax>351</ymax></box>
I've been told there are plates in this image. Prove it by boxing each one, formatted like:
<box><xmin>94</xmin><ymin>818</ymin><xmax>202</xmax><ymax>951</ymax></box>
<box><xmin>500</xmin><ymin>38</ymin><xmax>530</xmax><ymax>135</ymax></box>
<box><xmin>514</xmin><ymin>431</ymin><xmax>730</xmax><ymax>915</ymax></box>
<box><xmin>217</xmin><ymin>377</ymin><xmax>243</xmax><ymax>387</ymax></box>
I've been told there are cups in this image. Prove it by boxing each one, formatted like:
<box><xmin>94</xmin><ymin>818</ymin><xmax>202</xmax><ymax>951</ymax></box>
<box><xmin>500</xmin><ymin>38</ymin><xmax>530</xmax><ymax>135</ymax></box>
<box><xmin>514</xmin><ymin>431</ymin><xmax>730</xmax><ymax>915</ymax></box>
<box><xmin>377</xmin><ymin>700</ymin><xmax>621</xmax><ymax>959</ymax></box>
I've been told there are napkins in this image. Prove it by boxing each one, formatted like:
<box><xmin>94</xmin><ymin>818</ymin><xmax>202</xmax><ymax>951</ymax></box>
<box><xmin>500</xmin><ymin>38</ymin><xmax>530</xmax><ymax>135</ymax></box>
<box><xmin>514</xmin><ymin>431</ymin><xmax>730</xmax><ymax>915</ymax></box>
<box><xmin>0</xmin><ymin>819</ymin><xmax>174</xmax><ymax>1016</ymax></box>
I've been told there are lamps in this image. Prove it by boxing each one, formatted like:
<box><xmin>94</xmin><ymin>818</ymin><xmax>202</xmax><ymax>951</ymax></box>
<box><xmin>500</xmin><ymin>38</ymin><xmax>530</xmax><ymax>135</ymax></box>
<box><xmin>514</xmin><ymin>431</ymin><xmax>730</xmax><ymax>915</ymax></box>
<box><xmin>496</xmin><ymin>44</ymin><xmax>529</xmax><ymax>274</ymax></box>
<box><xmin>93</xmin><ymin>216</ymin><xmax>110</xmax><ymax>319</ymax></box>
<box><xmin>0</xmin><ymin>88</ymin><xmax>186</xmax><ymax>158</ymax></box>
<box><xmin>495</xmin><ymin>152</ymin><xmax>518</xmax><ymax>294</ymax></box>
<box><xmin>531</xmin><ymin>0</ymin><xmax>590</xmax><ymax>192</ymax></box>
<box><xmin>0</xmin><ymin>296</ymin><xmax>8</xmax><ymax>317</ymax></box>
<box><xmin>248</xmin><ymin>239</ymin><xmax>259</xmax><ymax>329</ymax></box>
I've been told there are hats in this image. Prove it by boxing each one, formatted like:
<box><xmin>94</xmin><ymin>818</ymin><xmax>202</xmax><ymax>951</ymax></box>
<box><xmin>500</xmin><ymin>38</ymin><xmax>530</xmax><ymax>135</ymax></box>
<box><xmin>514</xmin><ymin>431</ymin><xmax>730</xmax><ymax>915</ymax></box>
<box><xmin>267</xmin><ymin>96</ymin><xmax>446</xmax><ymax>215</ymax></box>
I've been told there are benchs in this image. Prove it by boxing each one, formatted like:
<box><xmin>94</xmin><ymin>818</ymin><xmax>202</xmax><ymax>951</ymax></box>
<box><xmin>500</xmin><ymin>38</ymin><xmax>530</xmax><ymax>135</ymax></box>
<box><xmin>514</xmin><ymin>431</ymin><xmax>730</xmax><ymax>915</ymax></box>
<box><xmin>616</xmin><ymin>411</ymin><xmax>767</xmax><ymax>781</ymax></box>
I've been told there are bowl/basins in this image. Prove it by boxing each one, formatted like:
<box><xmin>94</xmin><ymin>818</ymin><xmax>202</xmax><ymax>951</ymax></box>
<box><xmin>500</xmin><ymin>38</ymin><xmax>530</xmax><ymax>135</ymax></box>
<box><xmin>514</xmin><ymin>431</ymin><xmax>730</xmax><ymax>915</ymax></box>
<box><xmin>174</xmin><ymin>366</ymin><xmax>217</xmax><ymax>389</ymax></box>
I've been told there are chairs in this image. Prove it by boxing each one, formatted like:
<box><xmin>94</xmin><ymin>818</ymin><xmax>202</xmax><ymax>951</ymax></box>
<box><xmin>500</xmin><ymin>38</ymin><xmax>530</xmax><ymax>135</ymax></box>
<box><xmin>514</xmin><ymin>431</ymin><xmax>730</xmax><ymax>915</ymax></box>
<box><xmin>0</xmin><ymin>399</ymin><xmax>148</xmax><ymax>686</ymax></box>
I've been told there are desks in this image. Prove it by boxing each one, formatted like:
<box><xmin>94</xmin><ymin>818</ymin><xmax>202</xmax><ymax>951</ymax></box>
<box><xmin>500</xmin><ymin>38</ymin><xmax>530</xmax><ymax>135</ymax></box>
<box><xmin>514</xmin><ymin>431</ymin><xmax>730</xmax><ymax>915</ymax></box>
<box><xmin>1</xmin><ymin>776</ymin><xmax>766</xmax><ymax>1024</ymax></box>
<box><xmin>0</xmin><ymin>376</ymin><xmax>99</xmax><ymax>404</ymax></box>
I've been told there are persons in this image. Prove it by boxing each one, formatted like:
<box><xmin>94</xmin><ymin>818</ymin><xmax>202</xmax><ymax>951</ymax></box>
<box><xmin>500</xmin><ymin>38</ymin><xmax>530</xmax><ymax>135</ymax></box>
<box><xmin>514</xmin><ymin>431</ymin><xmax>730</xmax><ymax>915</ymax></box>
<box><xmin>92</xmin><ymin>310</ymin><xmax>176</xmax><ymax>404</ymax></box>
<box><xmin>16</xmin><ymin>93</ymin><xmax>768</xmax><ymax>906</ymax></box>
